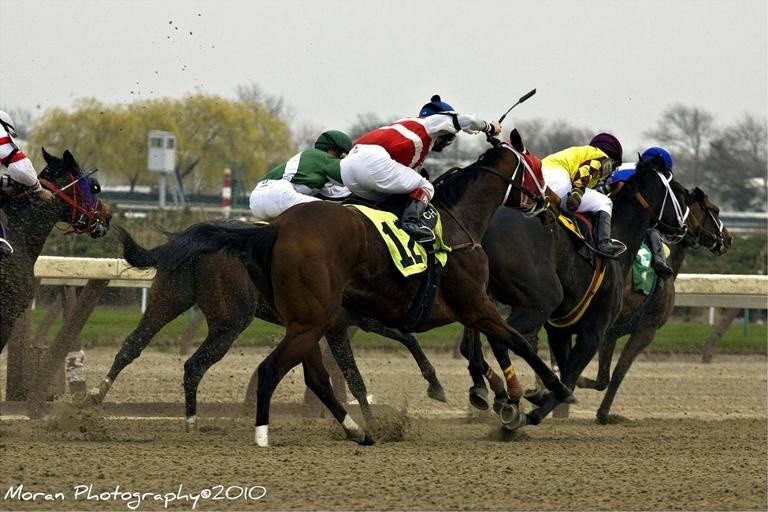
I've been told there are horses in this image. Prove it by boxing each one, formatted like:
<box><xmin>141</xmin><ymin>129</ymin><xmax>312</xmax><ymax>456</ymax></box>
<box><xmin>0</xmin><ymin>146</ymin><xmax>113</xmax><ymax>355</ymax></box>
<box><xmin>462</xmin><ymin>150</ymin><xmax>704</xmax><ymax>432</ymax></box>
<box><xmin>158</xmin><ymin>127</ymin><xmax>579</xmax><ymax>448</ymax></box>
<box><xmin>460</xmin><ymin>186</ymin><xmax>735</xmax><ymax>424</ymax></box>
<box><xmin>89</xmin><ymin>218</ymin><xmax>449</xmax><ymax>433</ymax></box>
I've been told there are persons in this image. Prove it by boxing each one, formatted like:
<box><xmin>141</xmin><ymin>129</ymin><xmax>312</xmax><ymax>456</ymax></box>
<box><xmin>249</xmin><ymin>130</ymin><xmax>351</xmax><ymax>223</ymax></box>
<box><xmin>1</xmin><ymin>108</ymin><xmax>56</xmax><ymax>260</ymax></box>
<box><xmin>539</xmin><ymin>133</ymin><xmax>626</xmax><ymax>256</ymax></box>
<box><xmin>339</xmin><ymin>98</ymin><xmax>503</xmax><ymax>240</ymax></box>
<box><xmin>592</xmin><ymin>148</ymin><xmax>674</xmax><ymax>279</ymax></box>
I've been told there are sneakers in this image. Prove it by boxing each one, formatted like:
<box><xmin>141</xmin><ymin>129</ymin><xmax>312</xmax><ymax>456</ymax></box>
<box><xmin>0</xmin><ymin>237</ymin><xmax>14</xmax><ymax>256</ymax></box>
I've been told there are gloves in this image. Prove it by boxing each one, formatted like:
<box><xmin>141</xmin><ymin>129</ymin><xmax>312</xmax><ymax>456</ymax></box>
<box><xmin>566</xmin><ymin>188</ymin><xmax>585</xmax><ymax>213</ymax></box>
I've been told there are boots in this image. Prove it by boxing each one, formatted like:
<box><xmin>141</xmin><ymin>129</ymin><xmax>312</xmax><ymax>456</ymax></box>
<box><xmin>644</xmin><ymin>229</ymin><xmax>673</xmax><ymax>276</ymax></box>
<box><xmin>402</xmin><ymin>189</ymin><xmax>436</xmax><ymax>238</ymax></box>
<box><xmin>593</xmin><ymin>210</ymin><xmax>625</xmax><ymax>254</ymax></box>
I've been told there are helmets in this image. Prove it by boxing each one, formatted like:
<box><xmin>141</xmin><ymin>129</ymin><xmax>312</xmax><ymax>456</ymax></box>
<box><xmin>589</xmin><ymin>133</ymin><xmax>622</xmax><ymax>166</ymax></box>
<box><xmin>315</xmin><ymin>130</ymin><xmax>351</xmax><ymax>154</ymax></box>
<box><xmin>417</xmin><ymin>95</ymin><xmax>455</xmax><ymax>119</ymax></box>
<box><xmin>1</xmin><ymin>110</ymin><xmax>16</xmax><ymax>136</ymax></box>
<box><xmin>641</xmin><ymin>147</ymin><xmax>672</xmax><ymax>170</ymax></box>
